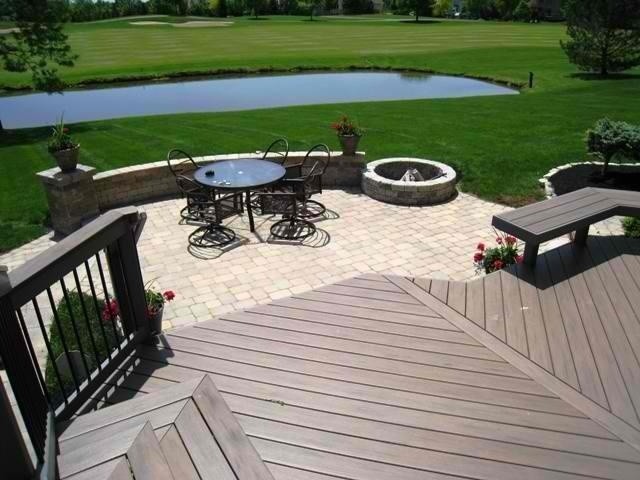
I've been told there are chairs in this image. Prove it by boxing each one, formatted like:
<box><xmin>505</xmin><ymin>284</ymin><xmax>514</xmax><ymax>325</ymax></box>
<box><xmin>167</xmin><ymin>137</ymin><xmax>331</xmax><ymax>247</ymax></box>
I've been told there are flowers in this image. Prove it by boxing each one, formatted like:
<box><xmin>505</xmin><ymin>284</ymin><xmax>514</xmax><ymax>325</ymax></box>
<box><xmin>102</xmin><ymin>275</ymin><xmax>176</xmax><ymax>322</ymax></box>
<box><xmin>45</xmin><ymin>109</ymin><xmax>75</xmax><ymax>153</ymax></box>
<box><xmin>472</xmin><ymin>226</ymin><xmax>550</xmax><ymax>277</ymax></box>
<box><xmin>327</xmin><ymin>112</ymin><xmax>365</xmax><ymax>137</ymax></box>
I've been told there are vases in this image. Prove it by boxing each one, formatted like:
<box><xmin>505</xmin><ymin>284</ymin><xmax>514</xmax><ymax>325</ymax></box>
<box><xmin>52</xmin><ymin>143</ymin><xmax>81</xmax><ymax>172</ymax></box>
<box><xmin>146</xmin><ymin>294</ymin><xmax>164</xmax><ymax>335</ymax></box>
<box><xmin>339</xmin><ymin>134</ymin><xmax>362</xmax><ymax>156</ymax></box>
